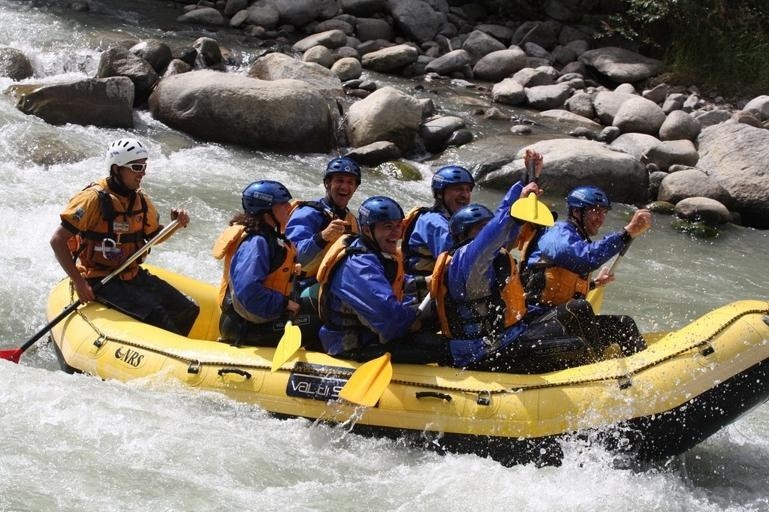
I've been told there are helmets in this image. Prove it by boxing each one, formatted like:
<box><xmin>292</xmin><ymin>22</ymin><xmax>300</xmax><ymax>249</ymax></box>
<box><xmin>431</xmin><ymin>166</ymin><xmax>475</xmax><ymax>189</ymax></box>
<box><xmin>450</xmin><ymin>202</ymin><xmax>495</xmax><ymax>237</ymax></box>
<box><xmin>242</xmin><ymin>179</ymin><xmax>293</xmax><ymax>215</ymax></box>
<box><xmin>567</xmin><ymin>185</ymin><xmax>612</xmax><ymax>207</ymax></box>
<box><xmin>106</xmin><ymin>137</ymin><xmax>148</xmax><ymax>173</ymax></box>
<box><xmin>356</xmin><ymin>195</ymin><xmax>406</xmax><ymax>226</ymax></box>
<box><xmin>324</xmin><ymin>159</ymin><xmax>362</xmax><ymax>184</ymax></box>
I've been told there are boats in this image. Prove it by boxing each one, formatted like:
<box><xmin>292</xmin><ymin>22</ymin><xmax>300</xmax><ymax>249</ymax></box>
<box><xmin>45</xmin><ymin>261</ymin><xmax>769</xmax><ymax>473</ymax></box>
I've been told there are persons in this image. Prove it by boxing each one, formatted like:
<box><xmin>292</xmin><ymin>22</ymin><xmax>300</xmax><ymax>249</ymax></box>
<box><xmin>399</xmin><ymin>166</ymin><xmax>475</xmax><ymax>301</ymax></box>
<box><xmin>283</xmin><ymin>158</ymin><xmax>362</xmax><ymax>302</ymax></box>
<box><xmin>317</xmin><ymin>196</ymin><xmax>455</xmax><ymax>367</ymax></box>
<box><xmin>430</xmin><ymin>149</ymin><xmax>543</xmax><ymax>373</ymax></box>
<box><xmin>211</xmin><ymin>180</ymin><xmax>324</xmax><ymax>353</ymax></box>
<box><xmin>50</xmin><ymin>139</ymin><xmax>199</xmax><ymax>337</ymax></box>
<box><xmin>519</xmin><ymin>186</ymin><xmax>652</xmax><ymax>358</ymax></box>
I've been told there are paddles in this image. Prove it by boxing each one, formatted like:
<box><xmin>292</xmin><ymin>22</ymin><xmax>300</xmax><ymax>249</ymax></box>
<box><xmin>338</xmin><ymin>291</ymin><xmax>431</xmax><ymax>407</ymax></box>
<box><xmin>0</xmin><ymin>218</ymin><xmax>181</xmax><ymax>364</ymax></box>
<box><xmin>509</xmin><ymin>157</ymin><xmax>554</xmax><ymax>228</ymax></box>
<box><xmin>271</xmin><ymin>270</ymin><xmax>303</xmax><ymax>371</ymax></box>
<box><xmin>586</xmin><ymin>236</ymin><xmax>636</xmax><ymax>314</ymax></box>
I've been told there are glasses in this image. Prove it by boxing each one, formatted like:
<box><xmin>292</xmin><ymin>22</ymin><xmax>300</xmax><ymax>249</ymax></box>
<box><xmin>121</xmin><ymin>164</ymin><xmax>148</xmax><ymax>172</ymax></box>
